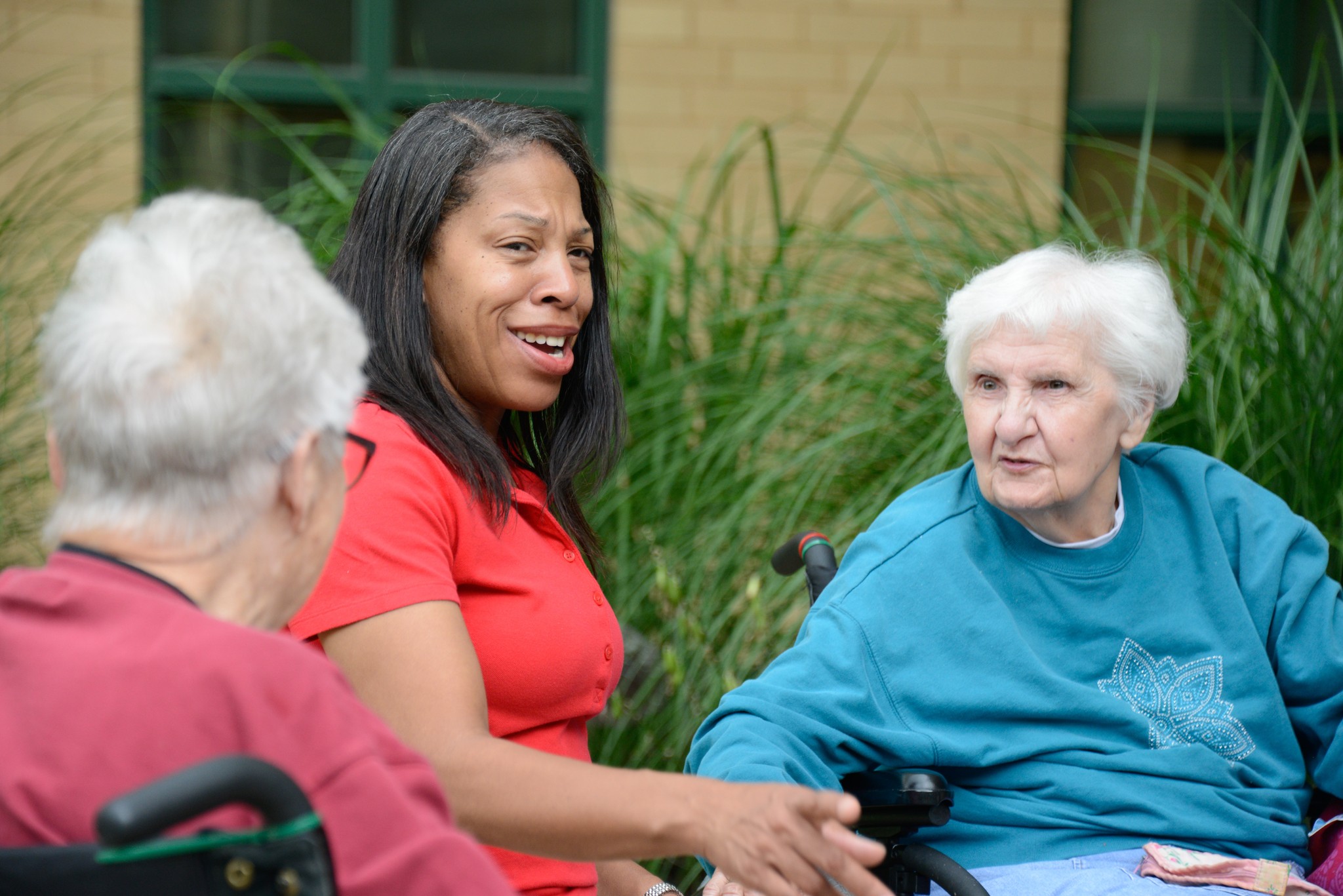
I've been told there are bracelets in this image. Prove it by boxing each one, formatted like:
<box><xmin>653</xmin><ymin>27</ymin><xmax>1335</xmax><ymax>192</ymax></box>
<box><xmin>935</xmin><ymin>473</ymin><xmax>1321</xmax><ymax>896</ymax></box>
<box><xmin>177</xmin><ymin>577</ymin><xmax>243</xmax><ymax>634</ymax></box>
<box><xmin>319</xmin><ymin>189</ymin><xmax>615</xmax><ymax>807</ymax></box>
<box><xmin>643</xmin><ymin>883</ymin><xmax>682</xmax><ymax>896</ymax></box>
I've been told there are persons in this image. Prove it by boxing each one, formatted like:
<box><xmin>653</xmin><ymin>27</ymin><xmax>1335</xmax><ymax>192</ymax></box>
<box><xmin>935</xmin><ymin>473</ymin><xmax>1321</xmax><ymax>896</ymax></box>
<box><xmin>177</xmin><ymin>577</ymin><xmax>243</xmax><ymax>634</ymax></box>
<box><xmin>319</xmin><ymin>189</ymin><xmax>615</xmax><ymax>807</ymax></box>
<box><xmin>0</xmin><ymin>193</ymin><xmax>528</xmax><ymax>896</ymax></box>
<box><xmin>281</xmin><ymin>98</ymin><xmax>897</xmax><ymax>896</ymax></box>
<box><xmin>683</xmin><ymin>243</ymin><xmax>1343</xmax><ymax>896</ymax></box>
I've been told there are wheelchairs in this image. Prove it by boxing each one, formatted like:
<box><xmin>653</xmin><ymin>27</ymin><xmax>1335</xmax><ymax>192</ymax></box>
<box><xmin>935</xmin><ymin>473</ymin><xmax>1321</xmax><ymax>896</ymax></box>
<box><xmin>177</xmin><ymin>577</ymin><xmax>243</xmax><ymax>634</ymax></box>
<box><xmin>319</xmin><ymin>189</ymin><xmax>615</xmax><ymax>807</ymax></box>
<box><xmin>771</xmin><ymin>531</ymin><xmax>1343</xmax><ymax>896</ymax></box>
<box><xmin>0</xmin><ymin>754</ymin><xmax>338</xmax><ymax>896</ymax></box>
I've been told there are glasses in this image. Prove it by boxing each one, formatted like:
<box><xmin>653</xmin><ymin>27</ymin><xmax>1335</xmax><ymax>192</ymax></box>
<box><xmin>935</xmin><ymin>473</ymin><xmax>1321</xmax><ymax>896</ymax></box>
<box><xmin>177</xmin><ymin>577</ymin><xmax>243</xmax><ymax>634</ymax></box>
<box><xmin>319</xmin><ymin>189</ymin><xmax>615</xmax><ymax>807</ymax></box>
<box><xmin>343</xmin><ymin>431</ymin><xmax>377</xmax><ymax>491</ymax></box>
